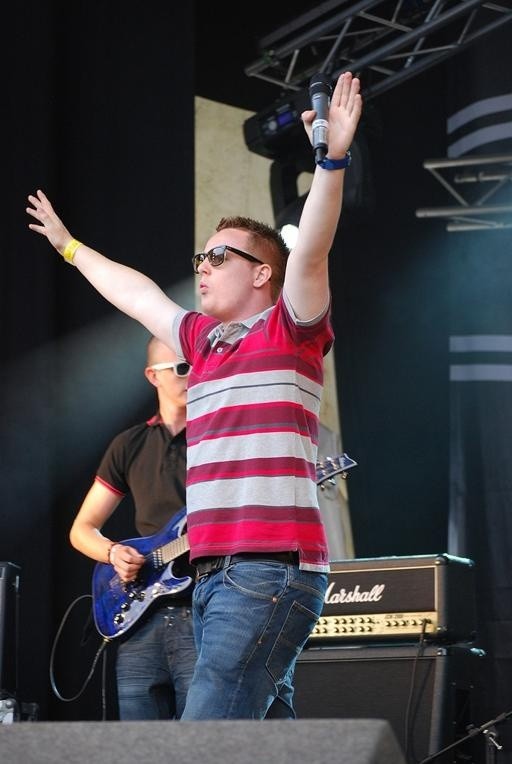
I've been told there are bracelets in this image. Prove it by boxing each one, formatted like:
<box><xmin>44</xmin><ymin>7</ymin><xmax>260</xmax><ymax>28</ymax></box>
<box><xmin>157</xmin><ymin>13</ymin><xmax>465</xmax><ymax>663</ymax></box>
<box><xmin>315</xmin><ymin>149</ymin><xmax>352</xmax><ymax>171</ymax></box>
<box><xmin>108</xmin><ymin>541</ymin><xmax>117</xmax><ymax>566</ymax></box>
<box><xmin>63</xmin><ymin>238</ymin><xmax>82</xmax><ymax>263</ymax></box>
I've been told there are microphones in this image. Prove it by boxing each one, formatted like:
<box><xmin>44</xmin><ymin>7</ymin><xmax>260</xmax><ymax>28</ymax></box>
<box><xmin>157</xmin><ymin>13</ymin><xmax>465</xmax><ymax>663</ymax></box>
<box><xmin>308</xmin><ymin>73</ymin><xmax>335</xmax><ymax>164</ymax></box>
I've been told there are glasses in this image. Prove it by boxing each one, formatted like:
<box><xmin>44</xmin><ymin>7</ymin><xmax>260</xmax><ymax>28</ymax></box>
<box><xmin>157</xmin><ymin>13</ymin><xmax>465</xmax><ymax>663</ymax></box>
<box><xmin>191</xmin><ymin>243</ymin><xmax>264</xmax><ymax>275</ymax></box>
<box><xmin>153</xmin><ymin>362</ymin><xmax>191</xmax><ymax>377</ymax></box>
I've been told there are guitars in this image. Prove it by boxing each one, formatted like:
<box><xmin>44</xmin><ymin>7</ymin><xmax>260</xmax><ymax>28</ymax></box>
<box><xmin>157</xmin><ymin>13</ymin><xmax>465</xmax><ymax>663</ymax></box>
<box><xmin>92</xmin><ymin>453</ymin><xmax>357</xmax><ymax>644</ymax></box>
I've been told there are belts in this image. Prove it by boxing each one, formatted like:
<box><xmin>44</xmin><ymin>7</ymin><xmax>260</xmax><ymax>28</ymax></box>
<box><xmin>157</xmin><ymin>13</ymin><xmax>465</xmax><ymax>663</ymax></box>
<box><xmin>193</xmin><ymin>547</ymin><xmax>301</xmax><ymax>583</ymax></box>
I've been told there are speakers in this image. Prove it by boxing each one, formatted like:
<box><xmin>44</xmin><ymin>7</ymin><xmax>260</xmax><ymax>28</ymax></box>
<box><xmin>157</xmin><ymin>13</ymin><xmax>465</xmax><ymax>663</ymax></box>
<box><xmin>0</xmin><ymin>717</ymin><xmax>407</xmax><ymax>763</ymax></box>
<box><xmin>290</xmin><ymin>644</ymin><xmax>490</xmax><ymax>764</ymax></box>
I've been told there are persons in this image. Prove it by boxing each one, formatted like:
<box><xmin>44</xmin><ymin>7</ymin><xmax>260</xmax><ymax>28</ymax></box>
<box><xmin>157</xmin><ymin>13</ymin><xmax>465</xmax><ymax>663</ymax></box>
<box><xmin>25</xmin><ymin>67</ymin><xmax>367</xmax><ymax>719</ymax></box>
<box><xmin>67</xmin><ymin>332</ymin><xmax>200</xmax><ymax>719</ymax></box>
